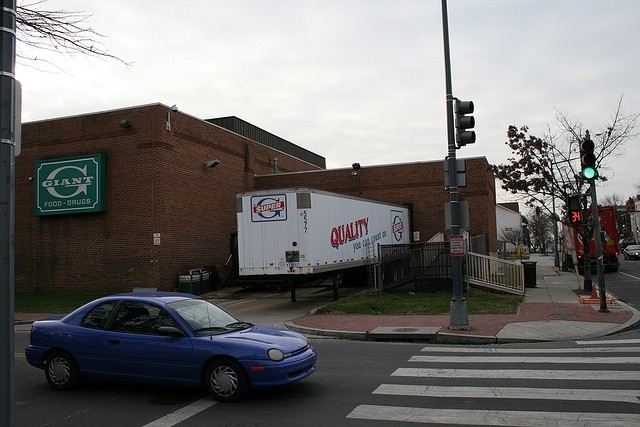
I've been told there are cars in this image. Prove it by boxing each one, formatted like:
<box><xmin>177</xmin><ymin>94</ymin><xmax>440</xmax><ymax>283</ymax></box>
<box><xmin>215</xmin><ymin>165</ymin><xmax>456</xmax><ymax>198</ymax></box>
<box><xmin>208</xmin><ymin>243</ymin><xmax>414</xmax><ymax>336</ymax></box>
<box><xmin>623</xmin><ymin>244</ymin><xmax>640</xmax><ymax>260</ymax></box>
<box><xmin>23</xmin><ymin>291</ymin><xmax>318</xmax><ymax>398</ymax></box>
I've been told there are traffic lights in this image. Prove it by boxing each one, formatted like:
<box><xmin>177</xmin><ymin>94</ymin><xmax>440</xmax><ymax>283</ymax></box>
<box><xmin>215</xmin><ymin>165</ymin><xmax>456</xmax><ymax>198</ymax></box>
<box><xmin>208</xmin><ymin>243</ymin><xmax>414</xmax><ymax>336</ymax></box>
<box><xmin>579</xmin><ymin>139</ymin><xmax>596</xmax><ymax>180</ymax></box>
<box><xmin>567</xmin><ymin>195</ymin><xmax>583</xmax><ymax>225</ymax></box>
<box><xmin>454</xmin><ymin>98</ymin><xmax>476</xmax><ymax>146</ymax></box>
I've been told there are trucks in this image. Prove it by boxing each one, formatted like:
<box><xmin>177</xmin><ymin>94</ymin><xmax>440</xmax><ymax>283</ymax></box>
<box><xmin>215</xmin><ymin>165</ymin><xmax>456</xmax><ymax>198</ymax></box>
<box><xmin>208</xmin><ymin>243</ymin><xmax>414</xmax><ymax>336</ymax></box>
<box><xmin>558</xmin><ymin>204</ymin><xmax>620</xmax><ymax>273</ymax></box>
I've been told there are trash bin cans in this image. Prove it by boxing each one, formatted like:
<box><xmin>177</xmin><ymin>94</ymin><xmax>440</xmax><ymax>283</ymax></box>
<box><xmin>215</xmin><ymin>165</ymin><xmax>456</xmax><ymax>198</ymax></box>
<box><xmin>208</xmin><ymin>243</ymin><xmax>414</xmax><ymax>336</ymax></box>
<box><xmin>202</xmin><ymin>266</ymin><xmax>218</xmax><ymax>293</ymax></box>
<box><xmin>520</xmin><ymin>261</ymin><xmax>537</xmax><ymax>289</ymax></box>
<box><xmin>178</xmin><ymin>268</ymin><xmax>202</xmax><ymax>295</ymax></box>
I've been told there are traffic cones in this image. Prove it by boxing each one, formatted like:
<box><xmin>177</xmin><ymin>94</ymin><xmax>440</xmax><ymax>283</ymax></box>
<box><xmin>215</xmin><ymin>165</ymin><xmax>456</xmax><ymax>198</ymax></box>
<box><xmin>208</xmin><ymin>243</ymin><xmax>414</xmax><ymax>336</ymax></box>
<box><xmin>590</xmin><ymin>284</ymin><xmax>600</xmax><ymax>299</ymax></box>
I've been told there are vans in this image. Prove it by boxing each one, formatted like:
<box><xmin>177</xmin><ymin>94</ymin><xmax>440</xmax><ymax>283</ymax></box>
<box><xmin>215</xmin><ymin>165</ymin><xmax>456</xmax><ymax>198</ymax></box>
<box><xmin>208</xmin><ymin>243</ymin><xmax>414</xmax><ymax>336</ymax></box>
<box><xmin>619</xmin><ymin>238</ymin><xmax>637</xmax><ymax>254</ymax></box>
